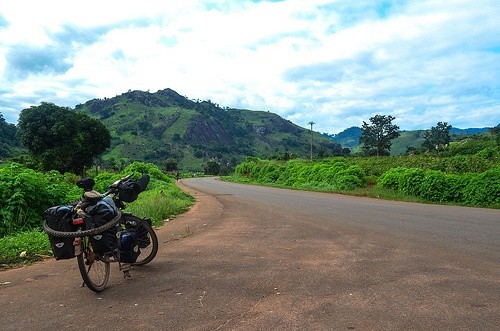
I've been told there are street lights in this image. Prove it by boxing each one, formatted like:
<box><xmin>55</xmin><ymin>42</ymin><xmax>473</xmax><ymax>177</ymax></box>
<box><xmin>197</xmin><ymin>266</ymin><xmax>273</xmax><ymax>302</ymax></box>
<box><xmin>308</xmin><ymin>121</ymin><xmax>316</xmax><ymax>161</ymax></box>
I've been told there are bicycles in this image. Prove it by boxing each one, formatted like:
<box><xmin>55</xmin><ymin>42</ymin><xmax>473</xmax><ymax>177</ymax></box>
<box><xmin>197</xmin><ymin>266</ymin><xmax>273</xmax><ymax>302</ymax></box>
<box><xmin>43</xmin><ymin>172</ymin><xmax>159</xmax><ymax>292</ymax></box>
